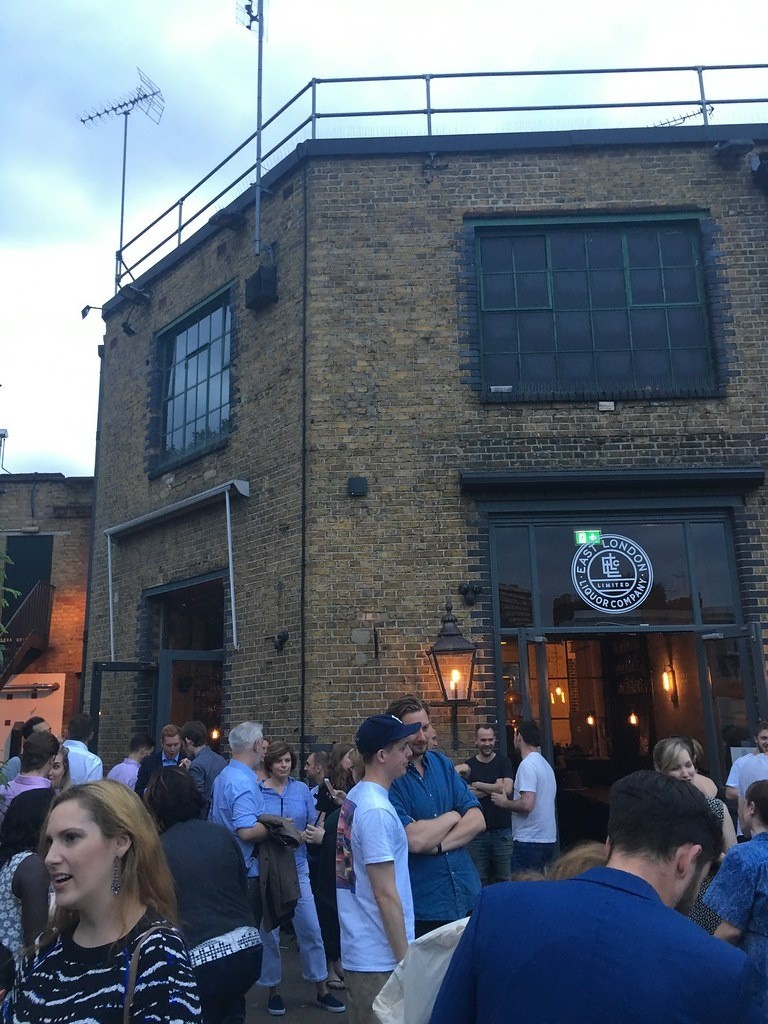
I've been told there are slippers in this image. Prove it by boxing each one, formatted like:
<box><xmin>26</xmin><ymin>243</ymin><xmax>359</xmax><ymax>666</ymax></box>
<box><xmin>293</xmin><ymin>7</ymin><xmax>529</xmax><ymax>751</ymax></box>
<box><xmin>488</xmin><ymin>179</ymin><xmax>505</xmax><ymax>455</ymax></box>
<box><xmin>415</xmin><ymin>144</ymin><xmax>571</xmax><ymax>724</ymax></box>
<box><xmin>326</xmin><ymin>977</ymin><xmax>347</xmax><ymax>990</ymax></box>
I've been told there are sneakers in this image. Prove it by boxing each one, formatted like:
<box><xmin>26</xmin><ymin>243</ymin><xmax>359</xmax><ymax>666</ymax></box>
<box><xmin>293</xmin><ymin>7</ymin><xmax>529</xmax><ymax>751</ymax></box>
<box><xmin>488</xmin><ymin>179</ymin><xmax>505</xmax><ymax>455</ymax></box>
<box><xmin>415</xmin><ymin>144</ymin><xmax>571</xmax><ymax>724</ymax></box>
<box><xmin>316</xmin><ymin>991</ymin><xmax>345</xmax><ymax>1012</ymax></box>
<box><xmin>267</xmin><ymin>993</ymin><xmax>286</xmax><ymax>1015</ymax></box>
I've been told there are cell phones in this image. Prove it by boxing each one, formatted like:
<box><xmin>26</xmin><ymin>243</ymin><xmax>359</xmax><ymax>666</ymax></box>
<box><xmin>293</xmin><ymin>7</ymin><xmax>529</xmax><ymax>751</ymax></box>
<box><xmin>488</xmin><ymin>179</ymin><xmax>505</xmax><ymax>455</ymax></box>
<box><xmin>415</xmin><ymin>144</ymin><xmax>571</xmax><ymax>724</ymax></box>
<box><xmin>324</xmin><ymin>779</ymin><xmax>336</xmax><ymax>798</ymax></box>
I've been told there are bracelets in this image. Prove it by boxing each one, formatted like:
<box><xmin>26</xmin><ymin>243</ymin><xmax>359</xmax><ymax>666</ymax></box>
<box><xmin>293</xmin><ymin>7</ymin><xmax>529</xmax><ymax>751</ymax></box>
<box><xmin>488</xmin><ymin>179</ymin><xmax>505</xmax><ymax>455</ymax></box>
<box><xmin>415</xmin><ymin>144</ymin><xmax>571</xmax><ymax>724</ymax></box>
<box><xmin>437</xmin><ymin>843</ymin><xmax>442</xmax><ymax>854</ymax></box>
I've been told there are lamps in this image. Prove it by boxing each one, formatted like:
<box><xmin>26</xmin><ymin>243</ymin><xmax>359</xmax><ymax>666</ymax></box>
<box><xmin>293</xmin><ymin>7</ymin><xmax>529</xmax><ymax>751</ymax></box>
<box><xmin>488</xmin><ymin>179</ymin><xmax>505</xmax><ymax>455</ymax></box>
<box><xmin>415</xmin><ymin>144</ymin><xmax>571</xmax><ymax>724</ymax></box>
<box><xmin>715</xmin><ymin>138</ymin><xmax>753</xmax><ymax>158</ymax></box>
<box><xmin>422</xmin><ymin>596</ymin><xmax>478</xmax><ymax>749</ymax></box>
<box><xmin>662</xmin><ymin>665</ymin><xmax>679</xmax><ymax>707</ymax></box>
<box><xmin>209</xmin><ymin>209</ymin><xmax>247</xmax><ymax>231</ymax></box>
<box><xmin>81</xmin><ymin>305</ymin><xmax>123</xmax><ymax>319</ymax></box>
<box><xmin>118</xmin><ymin>284</ymin><xmax>152</xmax><ymax>337</ymax></box>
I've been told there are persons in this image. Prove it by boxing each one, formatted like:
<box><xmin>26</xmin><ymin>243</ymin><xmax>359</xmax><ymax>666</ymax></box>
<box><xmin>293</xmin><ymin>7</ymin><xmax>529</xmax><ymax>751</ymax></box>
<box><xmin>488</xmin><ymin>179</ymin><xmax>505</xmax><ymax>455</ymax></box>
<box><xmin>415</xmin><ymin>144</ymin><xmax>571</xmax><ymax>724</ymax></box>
<box><xmin>653</xmin><ymin>722</ymin><xmax>768</xmax><ymax>977</ymax></box>
<box><xmin>337</xmin><ymin>716</ymin><xmax>422</xmax><ymax>1024</ymax></box>
<box><xmin>453</xmin><ymin>720</ymin><xmax>557</xmax><ymax>886</ymax></box>
<box><xmin>0</xmin><ymin>780</ymin><xmax>204</xmax><ymax>1024</ymax></box>
<box><xmin>386</xmin><ymin>696</ymin><xmax>486</xmax><ymax>939</ymax></box>
<box><xmin>428</xmin><ymin>770</ymin><xmax>768</xmax><ymax>1024</ymax></box>
<box><xmin>107</xmin><ymin>721</ymin><xmax>366</xmax><ymax>1024</ymax></box>
<box><xmin>0</xmin><ymin>714</ymin><xmax>103</xmax><ymax>1004</ymax></box>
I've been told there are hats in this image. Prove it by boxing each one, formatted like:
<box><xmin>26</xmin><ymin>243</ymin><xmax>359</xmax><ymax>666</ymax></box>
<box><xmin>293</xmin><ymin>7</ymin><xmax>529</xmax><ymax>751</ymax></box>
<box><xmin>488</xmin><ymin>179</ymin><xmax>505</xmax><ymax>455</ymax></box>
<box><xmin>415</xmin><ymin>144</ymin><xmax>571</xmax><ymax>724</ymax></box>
<box><xmin>356</xmin><ymin>715</ymin><xmax>422</xmax><ymax>757</ymax></box>
